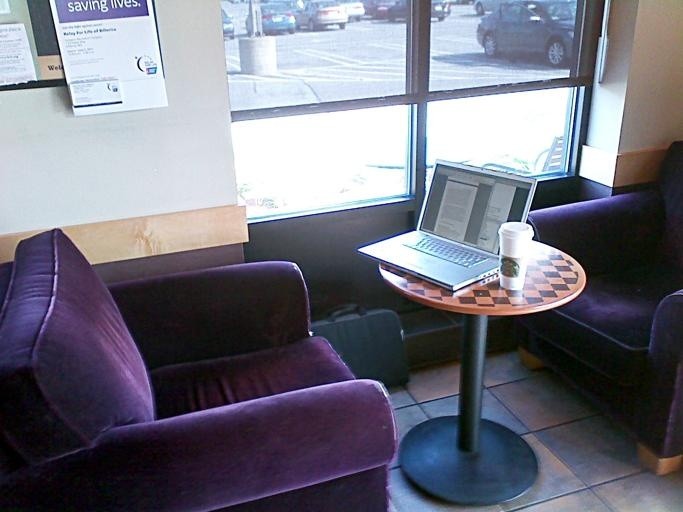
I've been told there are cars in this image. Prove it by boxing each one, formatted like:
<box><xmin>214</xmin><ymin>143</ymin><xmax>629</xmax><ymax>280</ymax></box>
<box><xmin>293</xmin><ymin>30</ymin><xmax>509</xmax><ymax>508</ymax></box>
<box><xmin>247</xmin><ymin>0</ymin><xmax>451</xmax><ymax>34</ymax></box>
<box><xmin>476</xmin><ymin>0</ymin><xmax>578</xmax><ymax>68</ymax></box>
<box><xmin>222</xmin><ymin>9</ymin><xmax>235</xmax><ymax>40</ymax></box>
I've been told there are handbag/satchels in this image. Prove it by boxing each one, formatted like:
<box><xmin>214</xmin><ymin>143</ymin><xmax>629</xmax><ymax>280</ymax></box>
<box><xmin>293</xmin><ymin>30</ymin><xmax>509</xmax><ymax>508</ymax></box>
<box><xmin>311</xmin><ymin>303</ymin><xmax>410</xmax><ymax>389</ymax></box>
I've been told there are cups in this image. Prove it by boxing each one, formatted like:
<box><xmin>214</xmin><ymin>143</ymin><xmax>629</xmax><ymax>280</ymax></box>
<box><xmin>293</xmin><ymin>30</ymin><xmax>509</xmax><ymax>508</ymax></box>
<box><xmin>498</xmin><ymin>222</ymin><xmax>535</xmax><ymax>291</ymax></box>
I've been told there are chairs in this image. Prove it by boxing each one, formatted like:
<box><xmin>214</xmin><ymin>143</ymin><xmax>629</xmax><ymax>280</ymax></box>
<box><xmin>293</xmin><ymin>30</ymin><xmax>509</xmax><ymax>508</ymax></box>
<box><xmin>524</xmin><ymin>139</ymin><xmax>683</xmax><ymax>477</ymax></box>
<box><xmin>0</xmin><ymin>229</ymin><xmax>399</xmax><ymax>512</ymax></box>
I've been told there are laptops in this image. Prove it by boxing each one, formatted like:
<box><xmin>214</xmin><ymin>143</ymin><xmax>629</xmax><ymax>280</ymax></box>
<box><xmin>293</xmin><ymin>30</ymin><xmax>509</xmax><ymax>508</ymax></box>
<box><xmin>357</xmin><ymin>158</ymin><xmax>538</xmax><ymax>292</ymax></box>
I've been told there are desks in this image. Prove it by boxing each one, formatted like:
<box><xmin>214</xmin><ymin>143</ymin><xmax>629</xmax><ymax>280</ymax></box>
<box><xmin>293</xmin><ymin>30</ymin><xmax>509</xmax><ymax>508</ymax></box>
<box><xmin>379</xmin><ymin>238</ymin><xmax>586</xmax><ymax>508</ymax></box>
